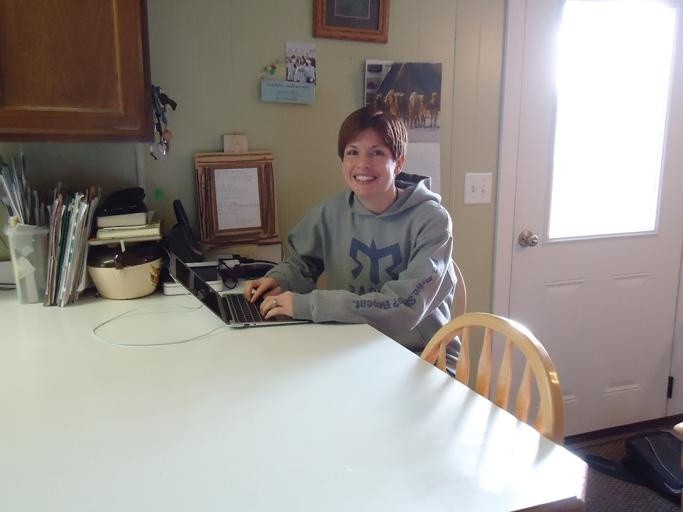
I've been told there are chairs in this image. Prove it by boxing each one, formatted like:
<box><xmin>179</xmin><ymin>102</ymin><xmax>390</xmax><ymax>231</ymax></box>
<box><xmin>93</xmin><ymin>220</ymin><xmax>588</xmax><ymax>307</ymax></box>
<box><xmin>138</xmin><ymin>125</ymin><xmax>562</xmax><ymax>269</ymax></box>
<box><xmin>419</xmin><ymin>312</ymin><xmax>566</xmax><ymax>447</ymax></box>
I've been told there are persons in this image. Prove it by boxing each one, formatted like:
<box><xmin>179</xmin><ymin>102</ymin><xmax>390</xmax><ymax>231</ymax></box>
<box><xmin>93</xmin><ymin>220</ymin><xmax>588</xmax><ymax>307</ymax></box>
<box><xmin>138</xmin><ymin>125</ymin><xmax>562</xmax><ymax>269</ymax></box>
<box><xmin>285</xmin><ymin>53</ymin><xmax>315</xmax><ymax>84</ymax></box>
<box><xmin>381</xmin><ymin>90</ymin><xmax>439</xmax><ymax>128</ymax></box>
<box><xmin>243</xmin><ymin>106</ymin><xmax>463</xmax><ymax>379</ymax></box>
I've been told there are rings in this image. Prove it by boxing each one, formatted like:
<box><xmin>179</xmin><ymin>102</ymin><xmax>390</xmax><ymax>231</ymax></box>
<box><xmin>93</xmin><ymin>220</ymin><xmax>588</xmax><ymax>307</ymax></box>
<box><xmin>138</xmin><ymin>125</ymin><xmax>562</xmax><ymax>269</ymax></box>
<box><xmin>272</xmin><ymin>299</ymin><xmax>277</xmax><ymax>307</ymax></box>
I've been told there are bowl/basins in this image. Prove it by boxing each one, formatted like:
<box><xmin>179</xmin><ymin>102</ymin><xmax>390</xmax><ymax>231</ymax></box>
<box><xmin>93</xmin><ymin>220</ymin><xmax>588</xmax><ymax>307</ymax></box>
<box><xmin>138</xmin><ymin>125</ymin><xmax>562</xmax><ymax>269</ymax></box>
<box><xmin>86</xmin><ymin>256</ymin><xmax>164</xmax><ymax>299</ymax></box>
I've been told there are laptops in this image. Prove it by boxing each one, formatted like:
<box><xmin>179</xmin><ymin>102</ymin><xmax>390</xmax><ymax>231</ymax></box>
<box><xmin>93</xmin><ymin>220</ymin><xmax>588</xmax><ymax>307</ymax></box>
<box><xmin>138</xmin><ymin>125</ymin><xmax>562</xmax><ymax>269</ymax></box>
<box><xmin>168</xmin><ymin>250</ymin><xmax>311</xmax><ymax>328</ymax></box>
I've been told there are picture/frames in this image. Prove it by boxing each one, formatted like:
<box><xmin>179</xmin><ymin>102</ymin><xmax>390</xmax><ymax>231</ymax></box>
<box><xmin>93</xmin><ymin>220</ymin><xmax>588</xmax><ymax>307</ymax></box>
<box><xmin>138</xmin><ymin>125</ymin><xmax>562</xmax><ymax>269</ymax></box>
<box><xmin>311</xmin><ymin>1</ymin><xmax>390</xmax><ymax>44</ymax></box>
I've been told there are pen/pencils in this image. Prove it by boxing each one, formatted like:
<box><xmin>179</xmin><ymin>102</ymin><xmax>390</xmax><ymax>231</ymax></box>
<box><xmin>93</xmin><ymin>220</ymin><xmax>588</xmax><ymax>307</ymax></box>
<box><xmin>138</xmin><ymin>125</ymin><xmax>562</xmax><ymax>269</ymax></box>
<box><xmin>218</xmin><ymin>254</ymin><xmax>240</xmax><ymax>259</ymax></box>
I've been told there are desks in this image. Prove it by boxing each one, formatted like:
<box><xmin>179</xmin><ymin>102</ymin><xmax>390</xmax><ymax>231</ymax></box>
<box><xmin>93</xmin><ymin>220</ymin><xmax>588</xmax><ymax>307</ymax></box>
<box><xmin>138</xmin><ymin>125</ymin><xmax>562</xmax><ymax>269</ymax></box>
<box><xmin>0</xmin><ymin>253</ymin><xmax>588</xmax><ymax>512</ymax></box>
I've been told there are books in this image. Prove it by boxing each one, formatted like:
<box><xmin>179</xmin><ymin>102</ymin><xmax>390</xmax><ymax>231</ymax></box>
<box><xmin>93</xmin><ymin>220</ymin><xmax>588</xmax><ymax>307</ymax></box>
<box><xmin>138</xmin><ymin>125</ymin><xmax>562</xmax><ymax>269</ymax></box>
<box><xmin>85</xmin><ymin>211</ymin><xmax>164</xmax><ymax>247</ymax></box>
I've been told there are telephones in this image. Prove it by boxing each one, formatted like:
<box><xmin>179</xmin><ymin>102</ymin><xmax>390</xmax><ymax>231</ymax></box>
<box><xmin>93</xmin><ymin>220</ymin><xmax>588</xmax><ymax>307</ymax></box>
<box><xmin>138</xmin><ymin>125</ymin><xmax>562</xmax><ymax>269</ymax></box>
<box><xmin>167</xmin><ymin>199</ymin><xmax>204</xmax><ymax>262</ymax></box>
